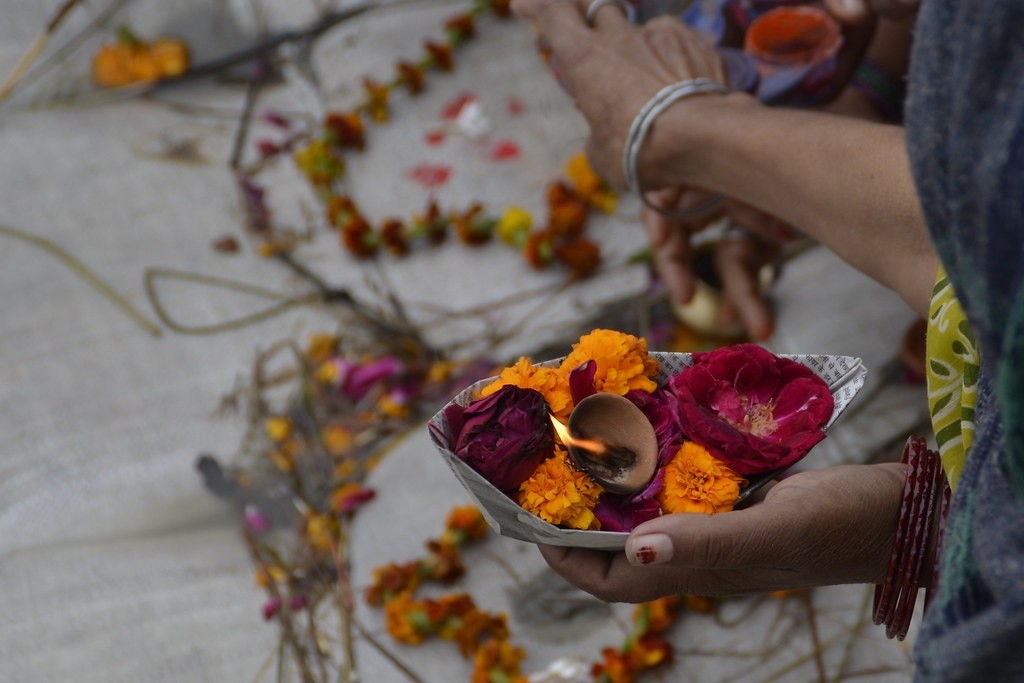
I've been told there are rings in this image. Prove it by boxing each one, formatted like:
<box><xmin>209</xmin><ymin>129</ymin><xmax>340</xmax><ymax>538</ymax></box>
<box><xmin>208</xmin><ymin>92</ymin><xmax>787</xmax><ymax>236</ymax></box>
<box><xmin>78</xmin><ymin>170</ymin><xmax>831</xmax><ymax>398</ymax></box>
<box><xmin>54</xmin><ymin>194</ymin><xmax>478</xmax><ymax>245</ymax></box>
<box><xmin>585</xmin><ymin>0</ymin><xmax>636</xmax><ymax>28</ymax></box>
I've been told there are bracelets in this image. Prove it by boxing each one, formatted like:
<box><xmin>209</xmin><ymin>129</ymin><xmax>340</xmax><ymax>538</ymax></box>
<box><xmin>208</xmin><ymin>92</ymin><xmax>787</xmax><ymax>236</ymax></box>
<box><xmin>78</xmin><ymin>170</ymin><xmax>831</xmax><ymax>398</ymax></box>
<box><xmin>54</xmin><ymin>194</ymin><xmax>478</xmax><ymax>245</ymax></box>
<box><xmin>872</xmin><ymin>432</ymin><xmax>951</xmax><ymax>642</ymax></box>
<box><xmin>622</xmin><ymin>77</ymin><xmax>732</xmax><ymax>218</ymax></box>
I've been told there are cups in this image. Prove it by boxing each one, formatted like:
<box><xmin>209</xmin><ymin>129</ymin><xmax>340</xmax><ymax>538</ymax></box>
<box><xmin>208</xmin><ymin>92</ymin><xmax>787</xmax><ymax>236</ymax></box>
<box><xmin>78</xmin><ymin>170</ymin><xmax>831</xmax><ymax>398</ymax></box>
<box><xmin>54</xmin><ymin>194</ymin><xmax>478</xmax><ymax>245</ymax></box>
<box><xmin>747</xmin><ymin>7</ymin><xmax>838</xmax><ymax>101</ymax></box>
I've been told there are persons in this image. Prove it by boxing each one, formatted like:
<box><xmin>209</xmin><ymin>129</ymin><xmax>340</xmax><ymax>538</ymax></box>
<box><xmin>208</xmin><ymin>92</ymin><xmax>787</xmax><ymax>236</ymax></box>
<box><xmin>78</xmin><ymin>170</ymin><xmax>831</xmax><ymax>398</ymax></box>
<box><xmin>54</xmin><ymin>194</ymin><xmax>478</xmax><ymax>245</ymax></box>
<box><xmin>509</xmin><ymin>0</ymin><xmax>1024</xmax><ymax>683</ymax></box>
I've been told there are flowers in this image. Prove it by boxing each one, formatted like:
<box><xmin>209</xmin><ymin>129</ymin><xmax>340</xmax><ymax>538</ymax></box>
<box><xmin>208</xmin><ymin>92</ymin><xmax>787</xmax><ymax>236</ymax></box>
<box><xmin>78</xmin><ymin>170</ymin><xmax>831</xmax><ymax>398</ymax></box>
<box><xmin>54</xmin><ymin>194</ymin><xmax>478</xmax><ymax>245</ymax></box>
<box><xmin>444</xmin><ymin>328</ymin><xmax>836</xmax><ymax>531</ymax></box>
